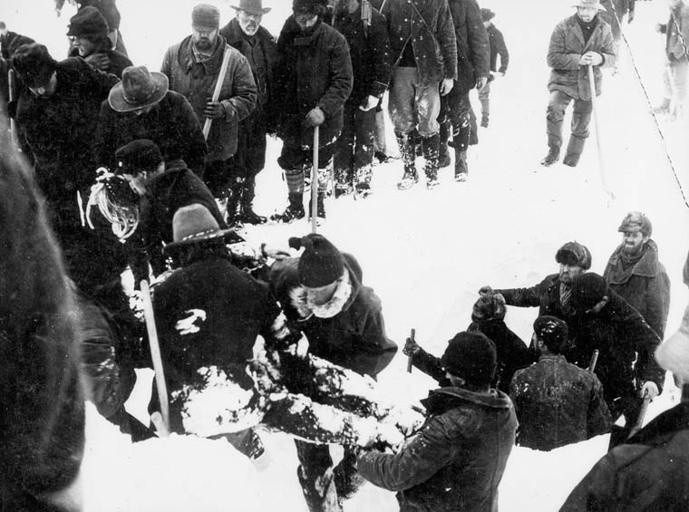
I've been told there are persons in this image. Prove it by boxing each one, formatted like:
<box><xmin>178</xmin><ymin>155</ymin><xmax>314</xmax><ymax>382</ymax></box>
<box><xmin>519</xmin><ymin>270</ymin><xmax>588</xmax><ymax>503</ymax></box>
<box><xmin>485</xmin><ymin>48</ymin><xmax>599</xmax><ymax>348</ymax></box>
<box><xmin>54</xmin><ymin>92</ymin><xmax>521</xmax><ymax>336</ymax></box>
<box><xmin>541</xmin><ymin>1</ymin><xmax>618</xmax><ymax>167</ymax></box>
<box><xmin>655</xmin><ymin>0</ymin><xmax>689</xmax><ymax>119</ymax></box>
<box><xmin>560</xmin><ymin>308</ymin><xmax>689</xmax><ymax>512</ymax></box>
<box><xmin>63</xmin><ymin>138</ymin><xmax>672</xmax><ymax>512</ymax></box>
<box><xmin>0</xmin><ymin>110</ymin><xmax>87</xmax><ymax>512</ymax></box>
<box><xmin>0</xmin><ymin>0</ymin><xmax>510</xmax><ymax>281</ymax></box>
<box><xmin>600</xmin><ymin>0</ymin><xmax>636</xmax><ymax>48</ymax></box>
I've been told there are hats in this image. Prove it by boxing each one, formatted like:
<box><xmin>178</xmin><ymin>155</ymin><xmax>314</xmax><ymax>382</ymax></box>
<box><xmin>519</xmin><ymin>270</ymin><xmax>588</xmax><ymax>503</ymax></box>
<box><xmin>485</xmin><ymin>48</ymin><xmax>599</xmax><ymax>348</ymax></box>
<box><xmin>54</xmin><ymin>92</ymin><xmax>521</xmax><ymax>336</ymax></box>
<box><xmin>471</xmin><ymin>294</ymin><xmax>506</xmax><ymax>329</ymax></box>
<box><xmin>441</xmin><ymin>330</ymin><xmax>499</xmax><ymax>388</ymax></box>
<box><xmin>535</xmin><ymin>316</ymin><xmax>570</xmax><ymax>355</ymax></box>
<box><xmin>480</xmin><ymin>7</ymin><xmax>495</xmax><ymax>22</ymax></box>
<box><xmin>571</xmin><ymin>0</ymin><xmax>607</xmax><ymax>11</ymax></box>
<box><xmin>617</xmin><ymin>212</ymin><xmax>653</xmax><ymax>236</ymax></box>
<box><xmin>555</xmin><ymin>239</ymin><xmax>592</xmax><ymax>273</ymax></box>
<box><xmin>230</xmin><ymin>0</ymin><xmax>273</xmax><ymax>14</ymax></box>
<box><xmin>292</xmin><ymin>0</ymin><xmax>331</xmax><ymax>15</ymax></box>
<box><xmin>108</xmin><ymin>66</ymin><xmax>170</xmax><ymax>115</ymax></box>
<box><xmin>289</xmin><ymin>234</ymin><xmax>345</xmax><ymax>288</ymax></box>
<box><xmin>191</xmin><ymin>3</ymin><xmax>220</xmax><ymax>34</ymax></box>
<box><xmin>162</xmin><ymin>203</ymin><xmax>240</xmax><ymax>253</ymax></box>
<box><xmin>12</xmin><ymin>43</ymin><xmax>58</xmax><ymax>89</ymax></box>
<box><xmin>570</xmin><ymin>271</ymin><xmax>607</xmax><ymax>313</ymax></box>
<box><xmin>67</xmin><ymin>6</ymin><xmax>108</xmax><ymax>36</ymax></box>
<box><xmin>115</xmin><ymin>139</ymin><xmax>163</xmax><ymax>175</ymax></box>
<box><xmin>271</xmin><ymin>165</ymin><xmax>326</xmax><ymax>230</ymax></box>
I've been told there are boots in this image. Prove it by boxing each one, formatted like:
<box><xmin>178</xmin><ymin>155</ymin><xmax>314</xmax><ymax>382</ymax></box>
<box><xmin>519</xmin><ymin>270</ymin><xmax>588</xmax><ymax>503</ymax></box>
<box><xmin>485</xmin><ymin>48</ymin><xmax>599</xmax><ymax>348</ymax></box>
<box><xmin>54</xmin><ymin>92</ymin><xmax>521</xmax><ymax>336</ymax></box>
<box><xmin>228</xmin><ymin>174</ymin><xmax>269</xmax><ymax>226</ymax></box>
<box><xmin>419</xmin><ymin>118</ymin><xmax>450</xmax><ymax>191</ymax></box>
<box><xmin>454</xmin><ymin>121</ymin><xmax>470</xmax><ymax>183</ymax></box>
<box><xmin>565</xmin><ymin>134</ymin><xmax>588</xmax><ymax>169</ymax></box>
<box><xmin>646</xmin><ymin>96</ymin><xmax>686</xmax><ymax>122</ymax></box>
<box><xmin>333</xmin><ymin>126</ymin><xmax>375</xmax><ymax>199</ymax></box>
<box><xmin>391</xmin><ymin>129</ymin><xmax>421</xmax><ymax>190</ymax></box>
<box><xmin>542</xmin><ymin>116</ymin><xmax>563</xmax><ymax>165</ymax></box>
